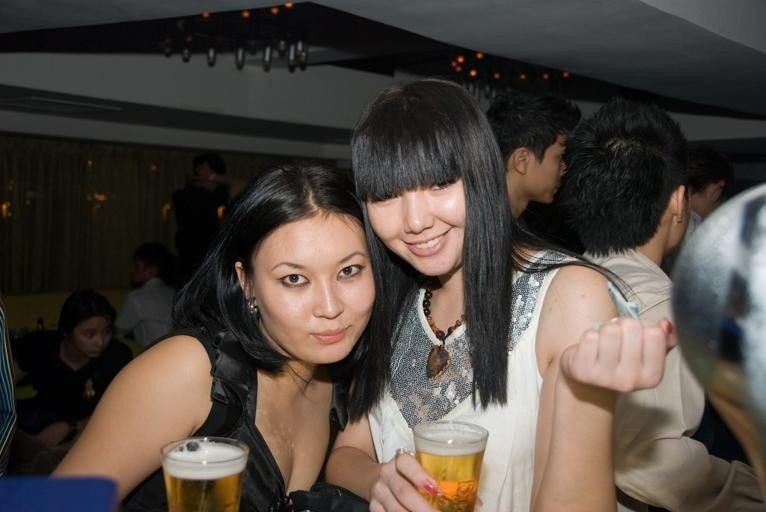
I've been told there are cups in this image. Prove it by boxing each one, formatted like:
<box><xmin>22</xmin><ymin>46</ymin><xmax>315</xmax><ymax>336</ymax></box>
<box><xmin>412</xmin><ymin>419</ymin><xmax>488</xmax><ymax>511</ymax></box>
<box><xmin>160</xmin><ymin>438</ymin><xmax>250</xmax><ymax>512</ymax></box>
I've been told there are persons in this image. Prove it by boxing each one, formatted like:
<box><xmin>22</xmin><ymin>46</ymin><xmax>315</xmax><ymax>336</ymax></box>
<box><xmin>2</xmin><ymin>80</ymin><xmax>764</xmax><ymax>509</ymax></box>
